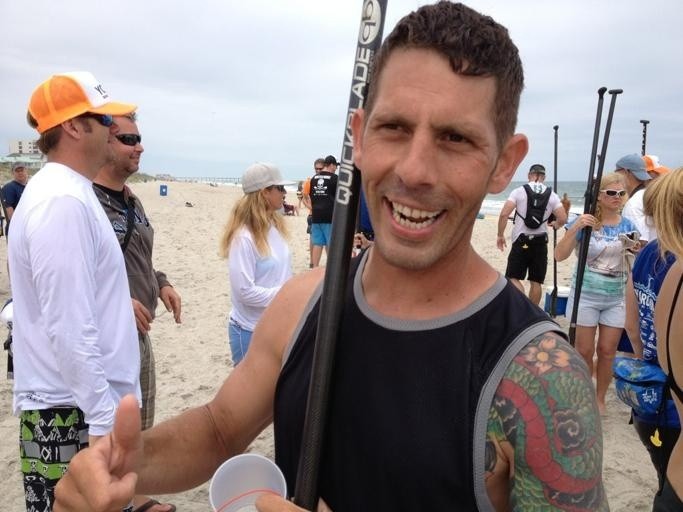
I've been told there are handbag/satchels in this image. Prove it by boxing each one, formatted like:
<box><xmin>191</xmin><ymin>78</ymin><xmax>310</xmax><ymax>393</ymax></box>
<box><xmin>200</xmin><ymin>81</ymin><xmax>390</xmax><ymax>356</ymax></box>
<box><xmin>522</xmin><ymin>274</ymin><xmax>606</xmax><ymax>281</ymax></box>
<box><xmin>611</xmin><ymin>356</ymin><xmax>669</xmax><ymax>415</ymax></box>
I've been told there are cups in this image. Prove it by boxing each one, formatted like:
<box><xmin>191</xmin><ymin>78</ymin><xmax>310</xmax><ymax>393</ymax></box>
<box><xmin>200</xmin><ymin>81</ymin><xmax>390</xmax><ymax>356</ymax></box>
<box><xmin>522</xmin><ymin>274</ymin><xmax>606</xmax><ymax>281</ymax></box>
<box><xmin>207</xmin><ymin>452</ymin><xmax>288</xmax><ymax>511</ymax></box>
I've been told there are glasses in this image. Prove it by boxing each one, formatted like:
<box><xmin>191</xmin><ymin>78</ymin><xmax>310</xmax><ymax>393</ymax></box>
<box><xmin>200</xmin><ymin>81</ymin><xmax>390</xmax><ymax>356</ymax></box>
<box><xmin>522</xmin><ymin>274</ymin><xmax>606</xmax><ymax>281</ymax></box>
<box><xmin>600</xmin><ymin>190</ymin><xmax>626</xmax><ymax>197</ymax></box>
<box><xmin>78</xmin><ymin>114</ymin><xmax>113</xmax><ymax>127</ymax></box>
<box><xmin>277</xmin><ymin>185</ymin><xmax>284</xmax><ymax>192</ymax></box>
<box><xmin>115</xmin><ymin>134</ymin><xmax>141</xmax><ymax>146</ymax></box>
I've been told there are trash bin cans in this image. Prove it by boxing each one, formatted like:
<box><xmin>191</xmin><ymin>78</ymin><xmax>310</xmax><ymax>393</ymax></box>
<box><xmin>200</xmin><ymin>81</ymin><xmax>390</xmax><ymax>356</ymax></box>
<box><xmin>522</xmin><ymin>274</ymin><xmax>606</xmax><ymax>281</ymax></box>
<box><xmin>160</xmin><ymin>185</ymin><xmax>167</xmax><ymax>196</ymax></box>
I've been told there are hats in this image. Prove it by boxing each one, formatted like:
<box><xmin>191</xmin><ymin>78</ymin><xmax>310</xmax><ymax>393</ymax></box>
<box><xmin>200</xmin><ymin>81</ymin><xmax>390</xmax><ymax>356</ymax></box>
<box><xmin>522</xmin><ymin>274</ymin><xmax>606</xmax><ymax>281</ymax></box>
<box><xmin>242</xmin><ymin>163</ymin><xmax>296</xmax><ymax>194</ymax></box>
<box><xmin>616</xmin><ymin>154</ymin><xmax>669</xmax><ymax>181</ymax></box>
<box><xmin>28</xmin><ymin>72</ymin><xmax>138</xmax><ymax>133</ymax></box>
<box><xmin>324</xmin><ymin>155</ymin><xmax>340</xmax><ymax>165</ymax></box>
<box><xmin>12</xmin><ymin>161</ymin><xmax>24</xmax><ymax>172</ymax></box>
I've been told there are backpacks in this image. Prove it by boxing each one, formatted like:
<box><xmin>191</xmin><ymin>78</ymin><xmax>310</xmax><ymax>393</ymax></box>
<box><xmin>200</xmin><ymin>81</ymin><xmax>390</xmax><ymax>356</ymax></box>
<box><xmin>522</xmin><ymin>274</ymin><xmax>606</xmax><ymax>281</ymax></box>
<box><xmin>523</xmin><ymin>184</ymin><xmax>551</xmax><ymax>229</ymax></box>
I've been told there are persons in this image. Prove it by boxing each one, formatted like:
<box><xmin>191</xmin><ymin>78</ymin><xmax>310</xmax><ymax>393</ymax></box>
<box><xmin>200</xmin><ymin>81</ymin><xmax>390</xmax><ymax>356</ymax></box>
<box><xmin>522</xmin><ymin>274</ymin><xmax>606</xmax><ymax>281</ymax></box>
<box><xmin>53</xmin><ymin>1</ymin><xmax>609</xmax><ymax>511</ymax></box>
<box><xmin>1</xmin><ymin>73</ymin><xmax>177</xmax><ymax>511</ymax></box>
<box><xmin>217</xmin><ymin>164</ymin><xmax>297</xmax><ymax>365</ymax></box>
<box><xmin>295</xmin><ymin>156</ymin><xmax>375</xmax><ymax>267</ymax></box>
<box><xmin>498</xmin><ymin>164</ymin><xmax>567</xmax><ymax>306</ymax></box>
<box><xmin>557</xmin><ymin>154</ymin><xmax>683</xmax><ymax>511</ymax></box>
